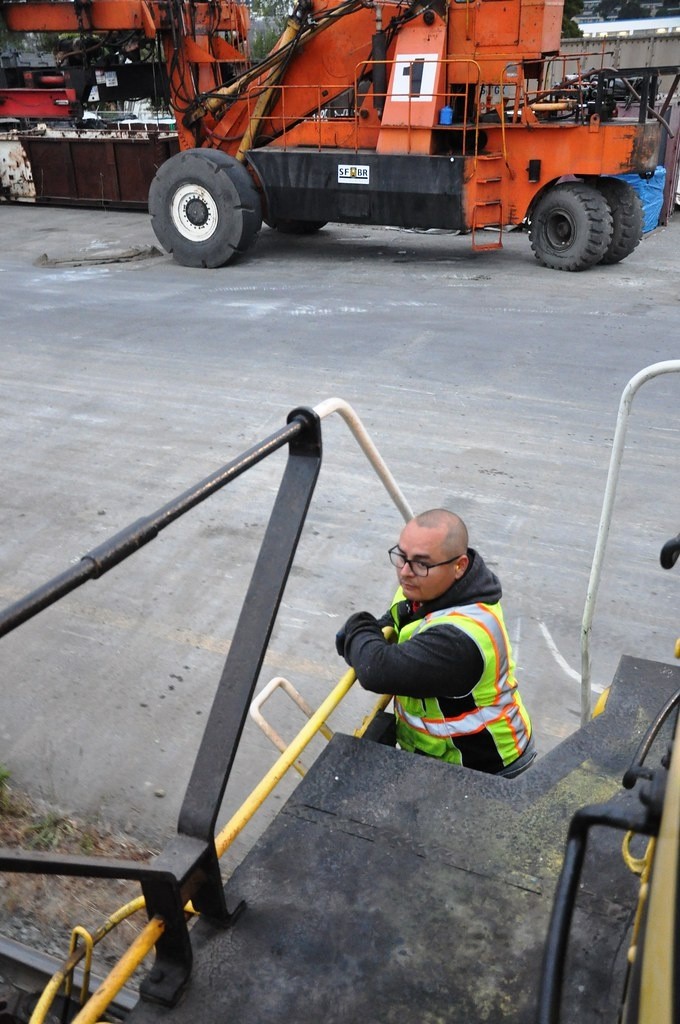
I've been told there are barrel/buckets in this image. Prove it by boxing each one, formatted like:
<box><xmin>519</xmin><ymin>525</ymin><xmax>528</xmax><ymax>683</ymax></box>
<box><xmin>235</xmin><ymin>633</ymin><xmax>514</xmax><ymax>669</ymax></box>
<box><xmin>440</xmin><ymin>105</ymin><xmax>453</xmax><ymax>125</ymax></box>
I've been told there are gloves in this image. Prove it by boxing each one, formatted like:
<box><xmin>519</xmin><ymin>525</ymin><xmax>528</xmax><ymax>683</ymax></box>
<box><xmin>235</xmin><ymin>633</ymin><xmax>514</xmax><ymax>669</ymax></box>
<box><xmin>336</xmin><ymin>630</ymin><xmax>346</xmax><ymax>657</ymax></box>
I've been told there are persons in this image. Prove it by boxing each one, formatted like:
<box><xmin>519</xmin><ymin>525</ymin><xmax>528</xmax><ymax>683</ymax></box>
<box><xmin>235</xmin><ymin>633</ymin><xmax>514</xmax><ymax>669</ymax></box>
<box><xmin>336</xmin><ymin>509</ymin><xmax>537</xmax><ymax>779</ymax></box>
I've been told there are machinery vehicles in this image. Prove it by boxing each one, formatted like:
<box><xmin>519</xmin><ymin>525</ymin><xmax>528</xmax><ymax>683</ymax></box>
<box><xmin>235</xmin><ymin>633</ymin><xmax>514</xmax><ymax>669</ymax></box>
<box><xmin>0</xmin><ymin>0</ymin><xmax>680</xmax><ymax>274</ymax></box>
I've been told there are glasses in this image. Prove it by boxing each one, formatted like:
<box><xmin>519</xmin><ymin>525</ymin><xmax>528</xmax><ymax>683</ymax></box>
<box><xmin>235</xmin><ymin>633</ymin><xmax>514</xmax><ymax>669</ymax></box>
<box><xmin>388</xmin><ymin>544</ymin><xmax>461</xmax><ymax>577</ymax></box>
